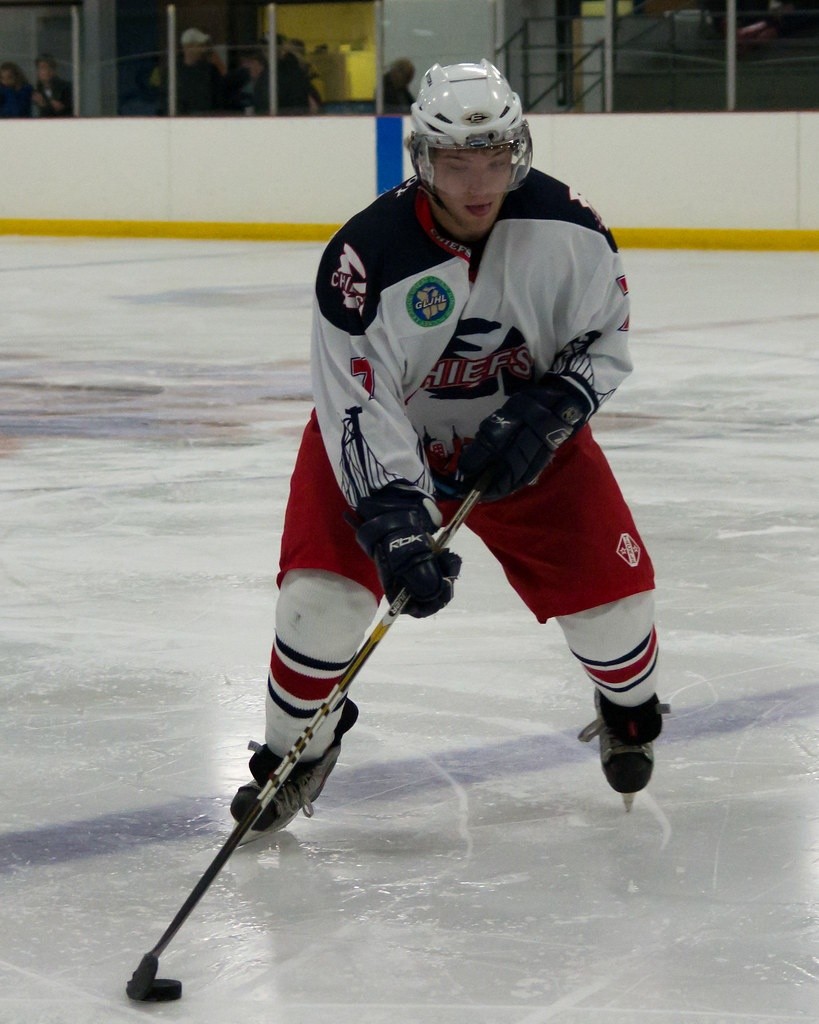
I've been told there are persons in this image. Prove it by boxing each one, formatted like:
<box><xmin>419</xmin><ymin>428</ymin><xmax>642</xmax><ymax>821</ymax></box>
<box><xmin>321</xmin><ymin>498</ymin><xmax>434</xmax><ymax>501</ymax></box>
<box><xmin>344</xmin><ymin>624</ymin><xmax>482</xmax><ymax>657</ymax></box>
<box><xmin>228</xmin><ymin>55</ymin><xmax>674</xmax><ymax>833</ymax></box>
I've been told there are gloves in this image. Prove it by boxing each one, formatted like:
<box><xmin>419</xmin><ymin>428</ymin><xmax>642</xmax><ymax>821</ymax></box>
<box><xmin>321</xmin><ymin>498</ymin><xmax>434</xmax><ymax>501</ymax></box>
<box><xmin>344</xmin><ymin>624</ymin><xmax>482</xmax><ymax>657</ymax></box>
<box><xmin>456</xmin><ymin>394</ymin><xmax>573</xmax><ymax>501</ymax></box>
<box><xmin>354</xmin><ymin>483</ymin><xmax>458</xmax><ymax>619</ymax></box>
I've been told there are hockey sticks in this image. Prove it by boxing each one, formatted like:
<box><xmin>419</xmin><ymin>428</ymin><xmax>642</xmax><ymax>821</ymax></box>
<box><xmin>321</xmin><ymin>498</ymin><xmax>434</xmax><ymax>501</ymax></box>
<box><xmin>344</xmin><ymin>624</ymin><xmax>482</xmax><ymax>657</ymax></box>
<box><xmin>128</xmin><ymin>477</ymin><xmax>482</xmax><ymax>997</ymax></box>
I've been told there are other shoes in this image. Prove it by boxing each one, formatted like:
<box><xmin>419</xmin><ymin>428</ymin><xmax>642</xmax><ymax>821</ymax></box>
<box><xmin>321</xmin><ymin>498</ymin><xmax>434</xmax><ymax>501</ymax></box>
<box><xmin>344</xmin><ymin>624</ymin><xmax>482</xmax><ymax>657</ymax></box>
<box><xmin>594</xmin><ymin>691</ymin><xmax>656</xmax><ymax>811</ymax></box>
<box><xmin>228</xmin><ymin>745</ymin><xmax>344</xmax><ymax>849</ymax></box>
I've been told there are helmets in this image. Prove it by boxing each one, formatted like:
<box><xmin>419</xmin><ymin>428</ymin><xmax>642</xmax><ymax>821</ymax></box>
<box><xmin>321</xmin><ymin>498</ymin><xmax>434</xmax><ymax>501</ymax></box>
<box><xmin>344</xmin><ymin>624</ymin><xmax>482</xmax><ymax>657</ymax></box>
<box><xmin>406</xmin><ymin>60</ymin><xmax>535</xmax><ymax>190</ymax></box>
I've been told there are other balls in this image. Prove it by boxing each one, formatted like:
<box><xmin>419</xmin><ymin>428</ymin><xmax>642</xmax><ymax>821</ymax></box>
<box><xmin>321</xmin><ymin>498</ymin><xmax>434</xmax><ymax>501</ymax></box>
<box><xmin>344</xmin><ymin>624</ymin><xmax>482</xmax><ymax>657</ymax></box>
<box><xmin>140</xmin><ymin>979</ymin><xmax>182</xmax><ymax>1002</ymax></box>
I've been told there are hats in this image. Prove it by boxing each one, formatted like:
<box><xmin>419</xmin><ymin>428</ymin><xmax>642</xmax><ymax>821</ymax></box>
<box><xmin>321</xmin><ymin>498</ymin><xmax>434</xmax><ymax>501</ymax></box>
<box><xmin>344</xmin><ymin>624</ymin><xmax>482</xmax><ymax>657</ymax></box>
<box><xmin>179</xmin><ymin>28</ymin><xmax>210</xmax><ymax>44</ymax></box>
